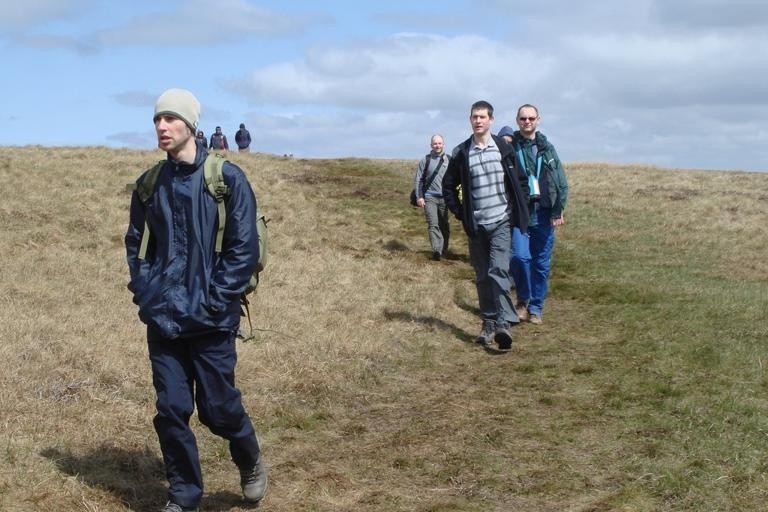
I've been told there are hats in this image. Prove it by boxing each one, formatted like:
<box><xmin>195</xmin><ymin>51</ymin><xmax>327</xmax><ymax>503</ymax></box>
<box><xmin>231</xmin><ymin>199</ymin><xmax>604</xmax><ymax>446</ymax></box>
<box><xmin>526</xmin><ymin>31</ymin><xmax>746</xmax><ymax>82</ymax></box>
<box><xmin>153</xmin><ymin>86</ymin><xmax>201</xmax><ymax>134</ymax></box>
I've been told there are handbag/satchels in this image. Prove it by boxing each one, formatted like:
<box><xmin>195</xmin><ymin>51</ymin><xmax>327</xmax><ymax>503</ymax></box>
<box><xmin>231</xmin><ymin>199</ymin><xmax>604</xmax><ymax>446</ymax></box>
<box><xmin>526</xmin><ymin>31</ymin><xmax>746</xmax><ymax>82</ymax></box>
<box><xmin>410</xmin><ymin>188</ymin><xmax>425</xmax><ymax>206</ymax></box>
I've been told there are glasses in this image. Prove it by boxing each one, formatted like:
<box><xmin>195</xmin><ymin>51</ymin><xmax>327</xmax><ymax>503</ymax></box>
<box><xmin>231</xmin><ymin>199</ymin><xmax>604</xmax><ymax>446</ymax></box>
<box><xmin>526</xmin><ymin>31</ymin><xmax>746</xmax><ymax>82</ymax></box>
<box><xmin>518</xmin><ymin>115</ymin><xmax>537</xmax><ymax>120</ymax></box>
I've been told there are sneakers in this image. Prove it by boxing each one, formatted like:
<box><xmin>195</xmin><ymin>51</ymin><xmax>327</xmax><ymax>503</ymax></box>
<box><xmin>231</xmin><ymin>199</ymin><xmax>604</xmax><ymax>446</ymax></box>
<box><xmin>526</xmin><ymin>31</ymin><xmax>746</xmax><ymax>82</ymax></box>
<box><xmin>515</xmin><ymin>299</ymin><xmax>528</xmax><ymax>320</ymax></box>
<box><xmin>529</xmin><ymin>311</ymin><xmax>542</xmax><ymax>325</ymax></box>
<box><xmin>475</xmin><ymin>321</ymin><xmax>495</xmax><ymax>344</ymax></box>
<box><xmin>432</xmin><ymin>248</ymin><xmax>449</xmax><ymax>261</ymax></box>
<box><xmin>237</xmin><ymin>452</ymin><xmax>267</xmax><ymax>503</ymax></box>
<box><xmin>160</xmin><ymin>501</ymin><xmax>188</xmax><ymax>512</ymax></box>
<box><xmin>495</xmin><ymin>321</ymin><xmax>511</xmax><ymax>349</ymax></box>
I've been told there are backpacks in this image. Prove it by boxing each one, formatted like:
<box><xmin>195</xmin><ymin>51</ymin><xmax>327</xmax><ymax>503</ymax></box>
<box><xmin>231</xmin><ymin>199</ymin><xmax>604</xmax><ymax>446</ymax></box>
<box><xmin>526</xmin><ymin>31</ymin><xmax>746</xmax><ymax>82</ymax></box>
<box><xmin>141</xmin><ymin>151</ymin><xmax>268</xmax><ymax>294</ymax></box>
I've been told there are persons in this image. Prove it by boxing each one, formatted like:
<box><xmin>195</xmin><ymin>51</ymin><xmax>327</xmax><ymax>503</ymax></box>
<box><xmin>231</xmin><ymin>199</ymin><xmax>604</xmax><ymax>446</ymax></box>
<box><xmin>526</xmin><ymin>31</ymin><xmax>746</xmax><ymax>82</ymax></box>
<box><xmin>511</xmin><ymin>104</ymin><xmax>569</xmax><ymax>325</ymax></box>
<box><xmin>124</xmin><ymin>86</ymin><xmax>269</xmax><ymax>512</ymax></box>
<box><xmin>414</xmin><ymin>133</ymin><xmax>452</xmax><ymax>260</ymax></box>
<box><xmin>497</xmin><ymin>125</ymin><xmax>514</xmax><ymax>142</ymax></box>
<box><xmin>441</xmin><ymin>100</ymin><xmax>532</xmax><ymax>351</ymax></box>
<box><xmin>209</xmin><ymin>126</ymin><xmax>229</xmax><ymax>150</ymax></box>
<box><xmin>196</xmin><ymin>131</ymin><xmax>207</xmax><ymax>148</ymax></box>
<box><xmin>235</xmin><ymin>123</ymin><xmax>252</xmax><ymax>152</ymax></box>
